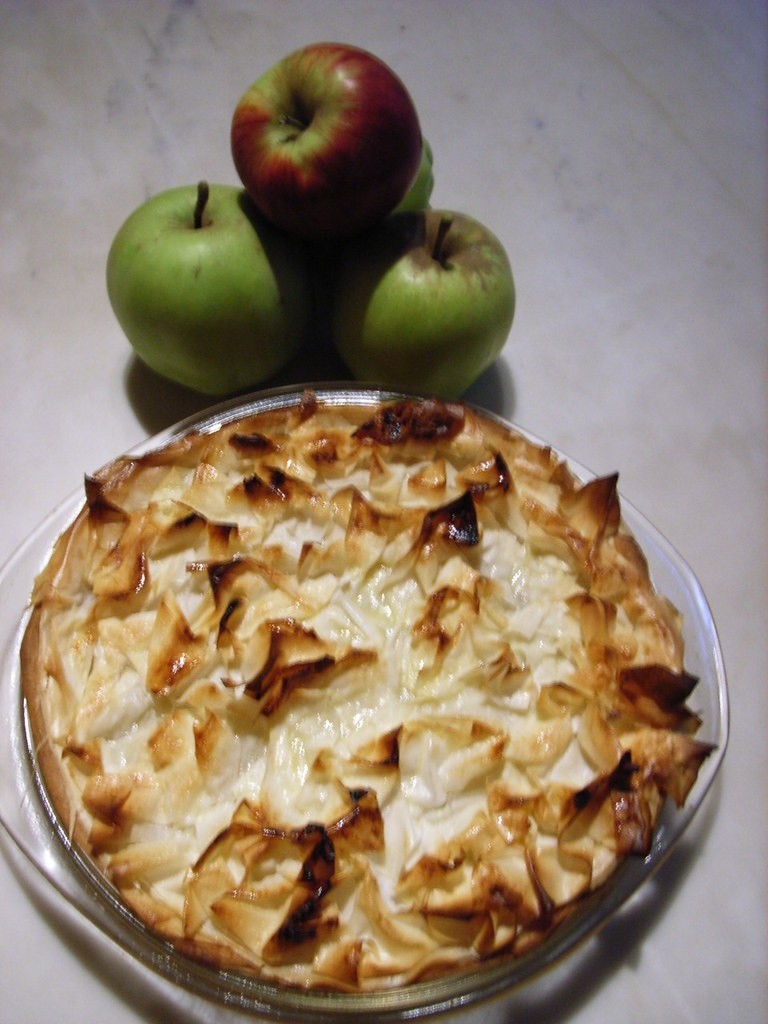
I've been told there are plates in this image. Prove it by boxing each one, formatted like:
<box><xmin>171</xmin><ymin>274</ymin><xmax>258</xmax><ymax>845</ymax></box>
<box><xmin>0</xmin><ymin>386</ymin><xmax>729</xmax><ymax>1022</ymax></box>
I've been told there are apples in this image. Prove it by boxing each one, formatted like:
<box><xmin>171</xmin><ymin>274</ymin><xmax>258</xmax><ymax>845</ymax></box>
<box><xmin>104</xmin><ymin>42</ymin><xmax>517</xmax><ymax>400</ymax></box>
